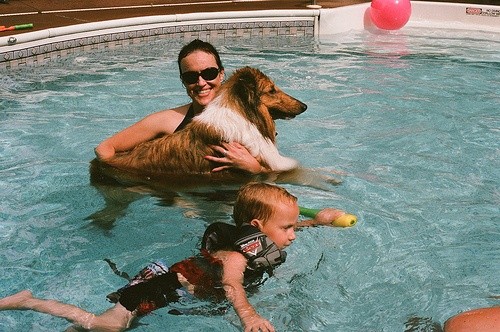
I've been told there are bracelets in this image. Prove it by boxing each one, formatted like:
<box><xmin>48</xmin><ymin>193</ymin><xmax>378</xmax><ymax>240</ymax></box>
<box><xmin>261</xmin><ymin>167</ymin><xmax>263</xmax><ymax>173</ymax></box>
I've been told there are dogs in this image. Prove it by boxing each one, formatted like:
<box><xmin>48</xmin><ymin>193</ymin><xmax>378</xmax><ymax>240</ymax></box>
<box><xmin>84</xmin><ymin>66</ymin><xmax>344</xmax><ymax>177</ymax></box>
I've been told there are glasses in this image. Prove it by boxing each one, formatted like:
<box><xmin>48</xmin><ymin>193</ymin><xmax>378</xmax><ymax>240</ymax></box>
<box><xmin>180</xmin><ymin>67</ymin><xmax>220</xmax><ymax>84</ymax></box>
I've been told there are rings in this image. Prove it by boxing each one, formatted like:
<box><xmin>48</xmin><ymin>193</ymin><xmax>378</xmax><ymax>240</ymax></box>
<box><xmin>223</xmin><ymin>150</ymin><xmax>226</xmax><ymax>156</ymax></box>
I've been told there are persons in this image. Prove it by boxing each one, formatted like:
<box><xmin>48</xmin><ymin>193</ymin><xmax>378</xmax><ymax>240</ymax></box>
<box><xmin>0</xmin><ymin>183</ymin><xmax>345</xmax><ymax>332</ymax></box>
<box><xmin>94</xmin><ymin>39</ymin><xmax>271</xmax><ymax>174</ymax></box>
<box><xmin>445</xmin><ymin>307</ymin><xmax>500</xmax><ymax>332</ymax></box>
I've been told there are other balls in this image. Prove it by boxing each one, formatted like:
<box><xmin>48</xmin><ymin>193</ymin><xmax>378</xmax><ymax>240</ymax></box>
<box><xmin>369</xmin><ymin>0</ymin><xmax>411</xmax><ymax>30</ymax></box>
